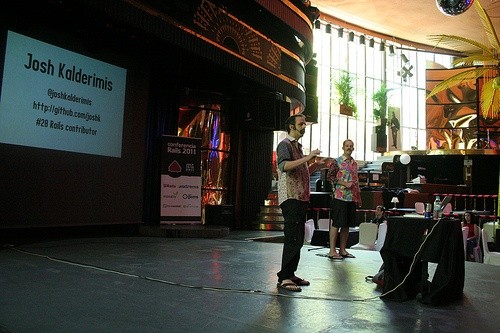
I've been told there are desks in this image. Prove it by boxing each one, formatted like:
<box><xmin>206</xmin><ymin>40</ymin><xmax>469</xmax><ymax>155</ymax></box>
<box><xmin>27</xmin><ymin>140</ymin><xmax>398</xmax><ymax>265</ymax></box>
<box><xmin>483</xmin><ymin>222</ymin><xmax>500</xmax><ymax>242</ymax></box>
<box><xmin>378</xmin><ymin>216</ymin><xmax>466</xmax><ymax>309</ymax></box>
<box><xmin>312</xmin><ymin>229</ymin><xmax>359</xmax><ymax>248</ymax></box>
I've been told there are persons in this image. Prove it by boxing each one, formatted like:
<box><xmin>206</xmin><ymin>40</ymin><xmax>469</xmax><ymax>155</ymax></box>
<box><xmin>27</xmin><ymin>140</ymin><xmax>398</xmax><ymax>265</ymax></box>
<box><xmin>324</xmin><ymin>140</ymin><xmax>362</xmax><ymax>260</ymax></box>
<box><xmin>372</xmin><ymin>206</ymin><xmax>386</xmax><ymax>241</ymax></box>
<box><xmin>460</xmin><ymin>211</ymin><xmax>477</xmax><ymax>256</ymax></box>
<box><xmin>275</xmin><ymin>115</ymin><xmax>335</xmax><ymax>292</ymax></box>
<box><xmin>391</xmin><ymin>112</ymin><xmax>401</xmax><ymax>150</ymax></box>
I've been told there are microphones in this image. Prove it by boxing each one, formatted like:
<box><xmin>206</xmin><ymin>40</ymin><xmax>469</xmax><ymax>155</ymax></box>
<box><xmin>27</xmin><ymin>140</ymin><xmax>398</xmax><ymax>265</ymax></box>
<box><xmin>293</xmin><ymin>127</ymin><xmax>304</xmax><ymax>134</ymax></box>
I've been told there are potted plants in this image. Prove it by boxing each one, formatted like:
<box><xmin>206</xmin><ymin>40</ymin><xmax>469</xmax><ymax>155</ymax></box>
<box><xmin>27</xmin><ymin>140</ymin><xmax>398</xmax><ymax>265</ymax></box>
<box><xmin>329</xmin><ymin>71</ymin><xmax>366</xmax><ymax>120</ymax></box>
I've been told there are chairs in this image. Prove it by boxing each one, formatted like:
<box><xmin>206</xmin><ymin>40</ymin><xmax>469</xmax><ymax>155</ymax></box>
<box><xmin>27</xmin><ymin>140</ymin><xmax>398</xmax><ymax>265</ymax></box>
<box><xmin>472</xmin><ymin>224</ymin><xmax>483</xmax><ymax>263</ymax></box>
<box><xmin>373</xmin><ymin>223</ymin><xmax>388</xmax><ymax>251</ymax></box>
<box><xmin>496</xmin><ymin>229</ymin><xmax>500</xmax><ymax>249</ymax></box>
<box><xmin>481</xmin><ymin>228</ymin><xmax>500</xmax><ymax>266</ymax></box>
<box><xmin>349</xmin><ymin>222</ymin><xmax>378</xmax><ymax>251</ymax></box>
<box><xmin>314</xmin><ymin>219</ymin><xmax>333</xmax><ymax>232</ymax></box>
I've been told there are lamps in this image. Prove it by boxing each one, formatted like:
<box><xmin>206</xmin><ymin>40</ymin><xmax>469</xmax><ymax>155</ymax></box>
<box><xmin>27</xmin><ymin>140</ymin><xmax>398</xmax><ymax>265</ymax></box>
<box><xmin>348</xmin><ymin>31</ymin><xmax>355</xmax><ymax>42</ymax></box>
<box><xmin>390</xmin><ymin>197</ymin><xmax>399</xmax><ymax>210</ymax></box>
<box><xmin>325</xmin><ymin>24</ymin><xmax>331</xmax><ymax>33</ymax></box>
<box><xmin>338</xmin><ymin>27</ymin><xmax>344</xmax><ymax>38</ymax></box>
<box><xmin>315</xmin><ymin>18</ymin><xmax>320</xmax><ymax>30</ymax></box>
<box><xmin>359</xmin><ymin>35</ymin><xmax>366</xmax><ymax>45</ymax></box>
<box><xmin>388</xmin><ymin>44</ymin><xmax>395</xmax><ymax>53</ymax></box>
<box><xmin>369</xmin><ymin>38</ymin><xmax>375</xmax><ymax>48</ymax></box>
<box><xmin>379</xmin><ymin>38</ymin><xmax>386</xmax><ymax>51</ymax></box>
<box><xmin>400</xmin><ymin>54</ymin><xmax>410</xmax><ymax>63</ymax></box>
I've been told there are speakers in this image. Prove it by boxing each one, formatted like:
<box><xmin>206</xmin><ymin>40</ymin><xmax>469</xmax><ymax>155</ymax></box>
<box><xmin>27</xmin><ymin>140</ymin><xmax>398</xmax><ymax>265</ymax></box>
<box><xmin>370</xmin><ymin>133</ymin><xmax>387</xmax><ymax>152</ymax></box>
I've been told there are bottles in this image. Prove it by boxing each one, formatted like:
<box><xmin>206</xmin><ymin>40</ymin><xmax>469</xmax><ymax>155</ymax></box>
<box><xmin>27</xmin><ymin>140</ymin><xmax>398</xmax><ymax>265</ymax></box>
<box><xmin>433</xmin><ymin>196</ymin><xmax>442</xmax><ymax>220</ymax></box>
<box><xmin>449</xmin><ymin>212</ymin><xmax>454</xmax><ymax>220</ymax></box>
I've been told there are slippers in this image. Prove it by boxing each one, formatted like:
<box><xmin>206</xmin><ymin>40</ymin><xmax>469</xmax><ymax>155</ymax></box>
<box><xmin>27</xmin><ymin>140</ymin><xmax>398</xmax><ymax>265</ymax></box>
<box><xmin>343</xmin><ymin>254</ymin><xmax>355</xmax><ymax>259</ymax></box>
<box><xmin>277</xmin><ymin>283</ymin><xmax>301</xmax><ymax>292</ymax></box>
<box><xmin>328</xmin><ymin>255</ymin><xmax>343</xmax><ymax>259</ymax></box>
<box><xmin>293</xmin><ymin>279</ymin><xmax>310</xmax><ymax>286</ymax></box>
